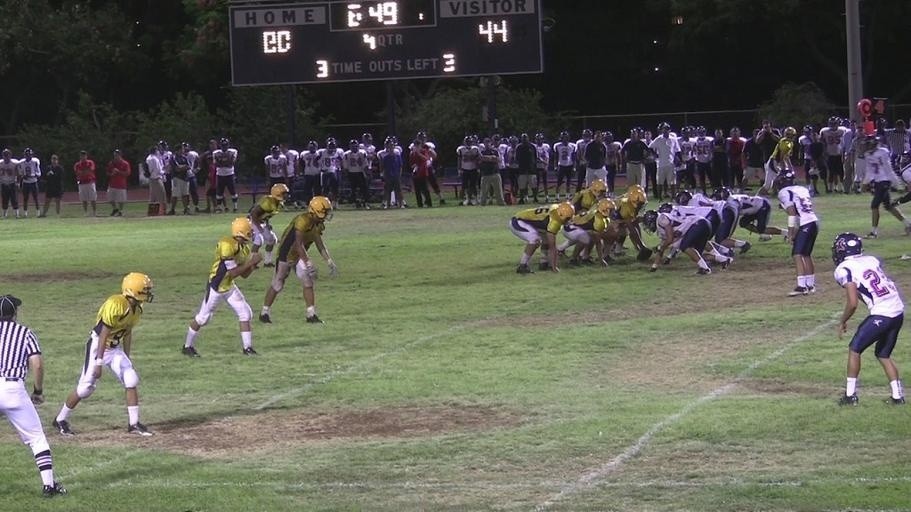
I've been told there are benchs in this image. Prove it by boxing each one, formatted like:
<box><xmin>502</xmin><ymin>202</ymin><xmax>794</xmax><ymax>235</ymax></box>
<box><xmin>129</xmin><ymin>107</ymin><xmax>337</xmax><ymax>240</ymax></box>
<box><xmin>442</xmin><ymin>178</ymin><xmax>588</xmax><ymax>198</ymax></box>
<box><xmin>244</xmin><ymin>186</ymin><xmax>409</xmax><ymax>213</ymax></box>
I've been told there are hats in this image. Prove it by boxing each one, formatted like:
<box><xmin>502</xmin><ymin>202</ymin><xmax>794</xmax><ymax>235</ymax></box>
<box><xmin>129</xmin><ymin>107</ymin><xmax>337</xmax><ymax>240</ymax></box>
<box><xmin>1</xmin><ymin>296</ymin><xmax>22</xmax><ymax>317</ymax></box>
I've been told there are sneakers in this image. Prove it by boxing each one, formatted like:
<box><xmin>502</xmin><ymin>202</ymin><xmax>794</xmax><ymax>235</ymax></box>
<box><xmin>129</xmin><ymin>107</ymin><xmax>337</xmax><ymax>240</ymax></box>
<box><xmin>181</xmin><ymin>313</ymin><xmax>323</xmax><ymax>358</ymax></box>
<box><xmin>128</xmin><ymin>422</ymin><xmax>155</xmax><ymax>436</ymax></box>
<box><xmin>43</xmin><ymin>480</ymin><xmax>67</xmax><ymax>498</ymax></box>
<box><xmin>838</xmin><ymin>391</ymin><xmax>859</xmax><ymax>406</ymax></box>
<box><xmin>53</xmin><ymin>416</ymin><xmax>75</xmax><ymax>437</ymax></box>
<box><xmin>883</xmin><ymin>397</ymin><xmax>905</xmax><ymax>405</ymax></box>
<box><xmin>863</xmin><ymin>233</ymin><xmax>877</xmax><ymax>238</ymax></box>
<box><xmin>516</xmin><ymin>237</ymin><xmax>771</xmax><ymax>274</ymax></box>
<box><xmin>787</xmin><ymin>285</ymin><xmax>816</xmax><ymax>297</ymax></box>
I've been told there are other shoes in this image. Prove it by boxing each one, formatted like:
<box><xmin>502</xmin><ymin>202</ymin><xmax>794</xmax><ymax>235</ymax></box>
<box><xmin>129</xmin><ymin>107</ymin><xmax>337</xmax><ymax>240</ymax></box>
<box><xmin>342</xmin><ymin>194</ymin><xmax>552</xmax><ymax>210</ymax></box>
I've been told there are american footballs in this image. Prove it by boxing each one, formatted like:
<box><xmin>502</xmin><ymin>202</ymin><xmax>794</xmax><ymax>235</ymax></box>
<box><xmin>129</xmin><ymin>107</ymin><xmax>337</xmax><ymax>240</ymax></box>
<box><xmin>637</xmin><ymin>248</ymin><xmax>652</xmax><ymax>261</ymax></box>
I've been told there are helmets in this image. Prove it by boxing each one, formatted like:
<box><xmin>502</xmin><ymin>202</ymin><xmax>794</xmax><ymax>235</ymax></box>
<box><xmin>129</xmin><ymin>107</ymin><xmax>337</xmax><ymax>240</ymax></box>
<box><xmin>308</xmin><ymin>140</ymin><xmax>319</xmax><ymax>151</ymax></box>
<box><xmin>556</xmin><ymin>179</ymin><xmax>732</xmax><ymax>235</ymax></box>
<box><xmin>271</xmin><ymin>146</ymin><xmax>280</xmax><ymax>155</ymax></box>
<box><xmin>153</xmin><ymin>136</ymin><xmax>230</xmax><ymax>151</ymax></box>
<box><xmin>832</xmin><ymin>232</ymin><xmax>862</xmax><ymax>265</ymax></box>
<box><xmin>230</xmin><ymin>182</ymin><xmax>333</xmax><ymax>242</ymax></box>
<box><xmin>580</xmin><ymin>128</ymin><xmax>614</xmax><ymax>146</ymax></box>
<box><xmin>773</xmin><ymin>170</ymin><xmax>797</xmax><ymax>190</ymax></box>
<box><xmin>463</xmin><ymin>132</ymin><xmax>545</xmax><ymax>147</ymax></box>
<box><xmin>560</xmin><ymin>132</ymin><xmax>569</xmax><ymax>140</ymax></box>
<box><xmin>121</xmin><ymin>272</ymin><xmax>154</xmax><ymax>303</ymax></box>
<box><xmin>326</xmin><ymin>137</ymin><xmax>337</xmax><ymax>155</ymax></box>
<box><xmin>417</xmin><ymin>132</ymin><xmax>427</xmax><ymax>140</ymax></box>
<box><xmin>363</xmin><ymin>133</ymin><xmax>373</xmax><ymax>147</ymax></box>
<box><xmin>384</xmin><ymin>136</ymin><xmax>398</xmax><ymax>147</ymax></box>
<box><xmin>629</xmin><ymin>123</ymin><xmax>905</xmax><ymax>153</ymax></box>
<box><xmin>349</xmin><ymin>140</ymin><xmax>359</xmax><ymax>149</ymax></box>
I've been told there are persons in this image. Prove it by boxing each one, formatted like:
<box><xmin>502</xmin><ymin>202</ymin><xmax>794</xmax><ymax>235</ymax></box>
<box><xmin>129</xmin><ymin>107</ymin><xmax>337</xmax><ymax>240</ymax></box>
<box><xmin>642</xmin><ymin>187</ymin><xmax>788</xmax><ymax>275</ymax></box>
<box><xmin>106</xmin><ymin>149</ymin><xmax>131</xmax><ymax>216</ymax></box>
<box><xmin>264</xmin><ymin>133</ymin><xmax>407</xmax><ymax>210</ymax></box>
<box><xmin>457</xmin><ymin>117</ymin><xmax>911</xmax><ymax>206</ymax></box>
<box><xmin>775</xmin><ymin>169</ymin><xmax>819</xmax><ymax>297</ymax></box>
<box><xmin>180</xmin><ymin>218</ymin><xmax>257</xmax><ymax>357</ymax></box>
<box><xmin>1</xmin><ymin>294</ymin><xmax>67</xmax><ymax>496</ymax></box>
<box><xmin>260</xmin><ymin>195</ymin><xmax>337</xmax><ymax>322</ymax></box>
<box><xmin>52</xmin><ymin>272</ymin><xmax>155</xmax><ymax>436</ymax></box>
<box><xmin>248</xmin><ymin>183</ymin><xmax>290</xmax><ymax>267</ymax></box>
<box><xmin>1</xmin><ymin>148</ymin><xmax>65</xmax><ymax>218</ymax></box>
<box><xmin>73</xmin><ymin>151</ymin><xmax>97</xmax><ymax>217</ymax></box>
<box><xmin>861</xmin><ymin>135</ymin><xmax>911</xmax><ymax>238</ymax></box>
<box><xmin>408</xmin><ymin>132</ymin><xmax>445</xmax><ymax>209</ymax></box>
<box><xmin>508</xmin><ymin>179</ymin><xmax>647</xmax><ymax>274</ymax></box>
<box><xmin>143</xmin><ymin>138</ymin><xmax>238</xmax><ymax>214</ymax></box>
<box><xmin>832</xmin><ymin>232</ymin><xmax>906</xmax><ymax>405</ymax></box>
